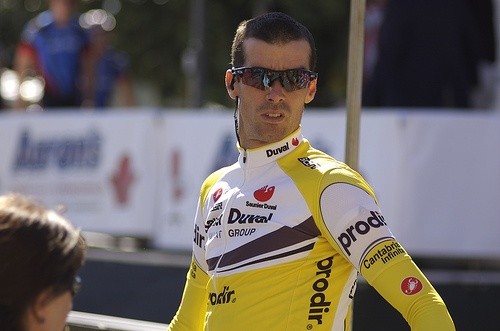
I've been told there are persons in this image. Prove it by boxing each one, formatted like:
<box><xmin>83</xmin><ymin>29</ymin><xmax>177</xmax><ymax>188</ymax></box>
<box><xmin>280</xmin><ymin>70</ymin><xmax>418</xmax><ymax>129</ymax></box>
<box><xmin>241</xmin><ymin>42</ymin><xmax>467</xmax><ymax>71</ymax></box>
<box><xmin>14</xmin><ymin>0</ymin><xmax>136</xmax><ymax>107</ymax></box>
<box><xmin>0</xmin><ymin>196</ymin><xmax>86</xmax><ymax>331</ymax></box>
<box><xmin>166</xmin><ymin>13</ymin><xmax>456</xmax><ymax>331</ymax></box>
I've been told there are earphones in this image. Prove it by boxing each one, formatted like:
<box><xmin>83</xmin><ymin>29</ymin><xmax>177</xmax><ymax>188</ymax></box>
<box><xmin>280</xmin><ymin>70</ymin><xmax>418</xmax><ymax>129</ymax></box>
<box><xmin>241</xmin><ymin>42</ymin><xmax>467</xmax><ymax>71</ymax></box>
<box><xmin>230</xmin><ymin>78</ymin><xmax>234</xmax><ymax>90</ymax></box>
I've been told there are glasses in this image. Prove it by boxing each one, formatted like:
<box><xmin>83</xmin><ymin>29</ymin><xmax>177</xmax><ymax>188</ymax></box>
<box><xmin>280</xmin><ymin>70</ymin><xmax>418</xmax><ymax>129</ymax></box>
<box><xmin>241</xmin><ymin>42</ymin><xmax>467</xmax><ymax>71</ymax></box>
<box><xmin>39</xmin><ymin>276</ymin><xmax>83</xmax><ymax>311</ymax></box>
<box><xmin>229</xmin><ymin>66</ymin><xmax>317</xmax><ymax>93</ymax></box>
